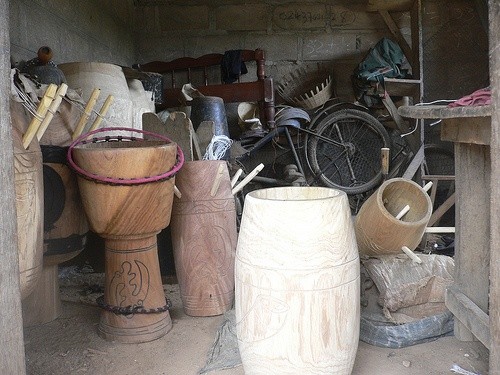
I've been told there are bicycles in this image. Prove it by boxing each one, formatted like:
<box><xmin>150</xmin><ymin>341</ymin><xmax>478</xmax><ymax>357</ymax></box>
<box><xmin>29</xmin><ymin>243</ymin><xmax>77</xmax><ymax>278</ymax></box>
<box><xmin>217</xmin><ymin>99</ymin><xmax>390</xmax><ymax>234</ymax></box>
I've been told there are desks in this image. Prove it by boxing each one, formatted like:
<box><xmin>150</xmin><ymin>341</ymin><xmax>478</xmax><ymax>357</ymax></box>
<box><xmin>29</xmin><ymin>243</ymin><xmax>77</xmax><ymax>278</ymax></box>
<box><xmin>399</xmin><ymin>99</ymin><xmax>492</xmax><ymax>352</ymax></box>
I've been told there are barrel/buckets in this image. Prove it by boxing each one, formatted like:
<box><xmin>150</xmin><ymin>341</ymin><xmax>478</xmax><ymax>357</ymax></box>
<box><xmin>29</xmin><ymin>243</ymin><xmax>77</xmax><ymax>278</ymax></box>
<box><xmin>171</xmin><ymin>160</ymin><xmax>237</xmax><ymax>316</ymax></box>
<box><xmin>235</xmin><ymin>186</ymin><xmax>360</xmax><ymax>375</ymax></box>
<box><xmin>56</xmin><ymin>61</ymin><xmax>134</xmax><ymax>141</ymax></box>
<box><xmin>354</xmin><ymin>178</ymin><xmax>434</xmax><ymax>257</ymax></box>
